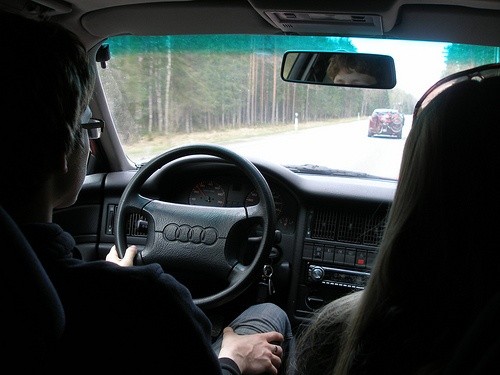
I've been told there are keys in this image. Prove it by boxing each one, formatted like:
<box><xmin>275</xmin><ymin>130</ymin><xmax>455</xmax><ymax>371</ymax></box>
<box><xmin>259</xmin><ymin>265</ymin><xmax>278</xmax><ymax>296</ymax></box>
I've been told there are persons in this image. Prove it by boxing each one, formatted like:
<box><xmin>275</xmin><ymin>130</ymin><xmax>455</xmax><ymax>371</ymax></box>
<box><xmin>327</xmin><ymin>53</ymin><xmax>393</xmax><ymax>87</ymax></box>
<box><xmin>0</xmin><ymin>13</ymin><xmax>297</xmax><ymax>375</ymax></box>
<box><xmin>298</xmin><ymin>64</ymin><xmax>500</xmax><ymax>375</ymax></box>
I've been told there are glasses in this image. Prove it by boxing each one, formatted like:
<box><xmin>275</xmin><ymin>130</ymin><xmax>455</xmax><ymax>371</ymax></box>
<box><xmin>413</xmin><ymin>63</ymin><xmax>500</xmax><ymax>125</ymax></box>
<box><xmin>79</xmin><ymin>118</ymin><xmax>105</xmax><ymax>140</ymax></box>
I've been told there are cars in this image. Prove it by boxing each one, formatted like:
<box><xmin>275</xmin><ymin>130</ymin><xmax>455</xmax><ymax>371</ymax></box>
<box><xmin>367</xmin><ymin>108</ymin><xmax>404</xmax><ymax>138</ymax></box>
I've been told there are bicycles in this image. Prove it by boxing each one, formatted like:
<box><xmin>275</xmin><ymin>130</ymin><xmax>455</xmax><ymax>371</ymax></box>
<box><xmin>373</xmin><ymin>110</ymin><xmax>404</xmax><ymax>136</ymax></box>
